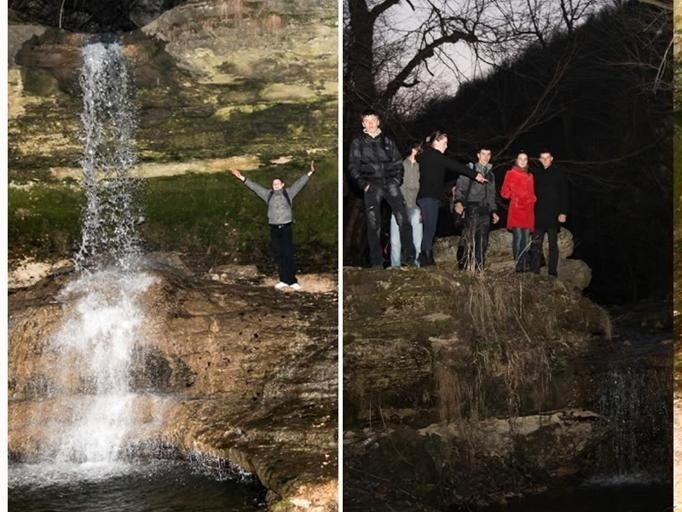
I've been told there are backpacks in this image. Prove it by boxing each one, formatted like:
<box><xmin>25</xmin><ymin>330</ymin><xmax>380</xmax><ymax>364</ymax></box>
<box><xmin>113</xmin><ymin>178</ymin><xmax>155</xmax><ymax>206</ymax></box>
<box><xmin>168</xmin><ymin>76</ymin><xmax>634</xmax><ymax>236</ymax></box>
<box><xmin>449</xmin><ymin>163</ymin><xmax>476</xmax><ymax>213</ymax></box>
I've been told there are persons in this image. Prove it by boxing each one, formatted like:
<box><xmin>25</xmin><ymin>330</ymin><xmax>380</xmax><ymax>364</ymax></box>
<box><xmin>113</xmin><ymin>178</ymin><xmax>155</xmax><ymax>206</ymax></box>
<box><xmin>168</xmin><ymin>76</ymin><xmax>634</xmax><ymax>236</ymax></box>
<box><xmin>532</xmin><ymin>148</ymin><xmax>567</xmax><ymax>277</ymax></box>
<box><xmin>346</xmin><ymin>108</ymin><xmax>419</xmax><ymax>271</ymax></box>
<box><xmin>453</xmin><ymin>143</ymin><xmax>500</xmax><ymax>272</ymax></box>
<box><xmin>415</xmin><ymin>130</ymin><xmax>488</xmax><ymax>267</ymax></box>
<box><xmin>389</xmin><ymin>140</ymin><xmax>424</xmax><ymax>269</ymax></box>
<box><xmin>231</xmin><ymin>159</ymin><xmax>315</xmax><ymax>291</ymax></box>
<box><xmin>498</xmin><ymin>148</ymin><xmax>537</xmax><ymax>261</ymax></box>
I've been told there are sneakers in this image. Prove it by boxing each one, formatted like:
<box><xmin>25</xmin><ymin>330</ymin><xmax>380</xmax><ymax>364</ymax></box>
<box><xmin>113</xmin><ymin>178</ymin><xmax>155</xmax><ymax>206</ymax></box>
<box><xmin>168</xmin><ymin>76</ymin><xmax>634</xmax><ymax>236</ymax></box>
<box><xmin>416</xmin><ymin>250</ymin><xmax>434</xmax><ymax>266</ymax></box>
<box><xmin>274</xmin><ymin>282</ymin><xmax>300</xmax><ymax>290</ymax></box>
